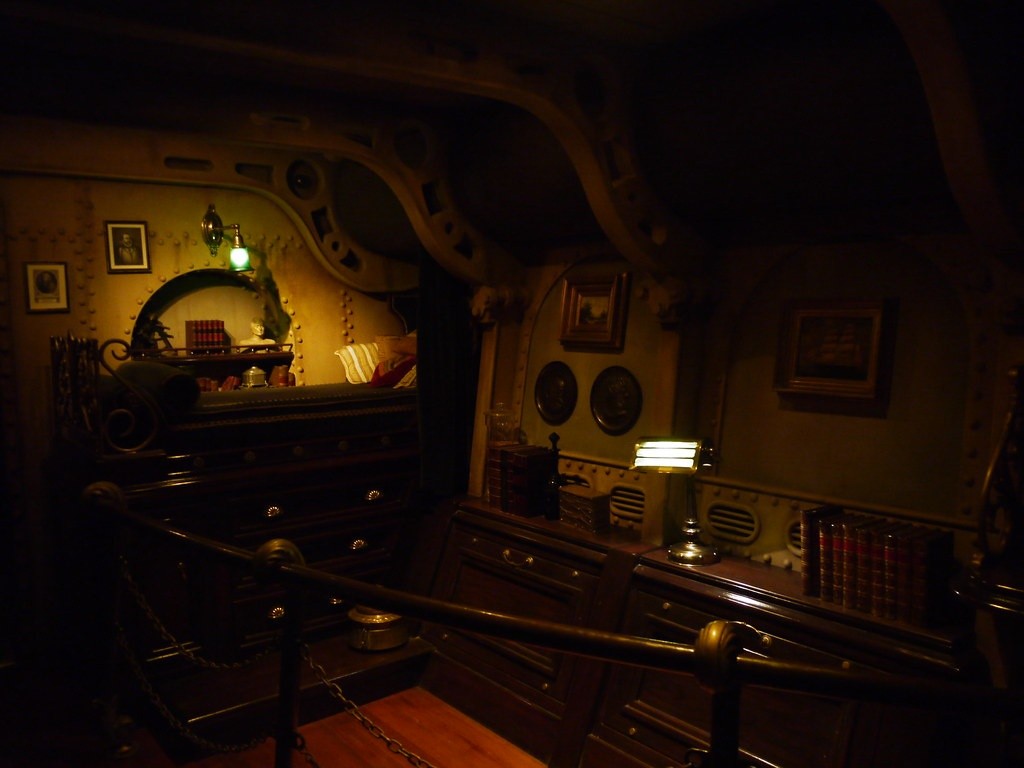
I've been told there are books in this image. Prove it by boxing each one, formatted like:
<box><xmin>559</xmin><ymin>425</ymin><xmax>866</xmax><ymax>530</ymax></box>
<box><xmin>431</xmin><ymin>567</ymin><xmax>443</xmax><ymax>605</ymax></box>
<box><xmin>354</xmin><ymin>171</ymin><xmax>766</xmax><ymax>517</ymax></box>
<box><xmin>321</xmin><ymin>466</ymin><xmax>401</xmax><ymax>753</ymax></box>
<box><xmin>800</xmin><ymin>503</ymin><xmax>955</xmax><ymax>628</ymax></box>
<box><xmin>487</xmin><ymin>445</ymin><xmax>553</xmax><ymax>520</ymax></box>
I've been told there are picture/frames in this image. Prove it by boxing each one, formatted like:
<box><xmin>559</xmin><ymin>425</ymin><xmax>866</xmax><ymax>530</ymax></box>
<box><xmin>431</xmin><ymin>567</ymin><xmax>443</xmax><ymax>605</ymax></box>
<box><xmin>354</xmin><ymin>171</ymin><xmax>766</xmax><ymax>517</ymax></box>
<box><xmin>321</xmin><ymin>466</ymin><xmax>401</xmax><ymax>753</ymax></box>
<box><xmin>557</xmin><ymin>270</ymin><xmax>629</xmax><ymax>355</ymax></box>
<box><xmin>772</xmin><ymin>294</ymin><xmax>901</xmax><ymax>420</ymax></box>
<box><xmin>102</xmin><ymin>219</ymin><xmax>153</xmax><ymax>275</ymax></box>
<box><xmin>22</xmin><ymin>261</ymin><xmax>70</xmax><ymax>315</ymax></box>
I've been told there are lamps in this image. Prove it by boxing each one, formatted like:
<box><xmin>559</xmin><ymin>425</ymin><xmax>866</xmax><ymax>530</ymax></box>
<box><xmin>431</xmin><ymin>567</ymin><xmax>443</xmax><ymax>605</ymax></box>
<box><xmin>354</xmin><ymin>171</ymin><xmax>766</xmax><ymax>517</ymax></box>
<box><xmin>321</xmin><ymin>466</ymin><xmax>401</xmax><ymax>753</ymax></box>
<box><xmin>200</xmin><ymin>202</ymin><xmax>255</xmax><ymax>274</ymax></box>
<box><xmin>628</xmin><ymin>436</ymin><xmax>721</xmax><ymax>567</ymax></box>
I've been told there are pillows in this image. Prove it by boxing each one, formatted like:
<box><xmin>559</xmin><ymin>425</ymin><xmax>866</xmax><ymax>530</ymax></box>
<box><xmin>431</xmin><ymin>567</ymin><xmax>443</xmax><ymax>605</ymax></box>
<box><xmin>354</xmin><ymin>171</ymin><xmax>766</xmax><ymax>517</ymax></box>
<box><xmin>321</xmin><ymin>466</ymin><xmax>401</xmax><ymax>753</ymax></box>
<box><xmin>333</xmin><ymin>341</ymin><xmax>379</xmax><ymax>385</ymax></box>
<box><xmin>375</xmin><ymin>334</ymin><xmax>416</xmax><ymax>376</ymax></box>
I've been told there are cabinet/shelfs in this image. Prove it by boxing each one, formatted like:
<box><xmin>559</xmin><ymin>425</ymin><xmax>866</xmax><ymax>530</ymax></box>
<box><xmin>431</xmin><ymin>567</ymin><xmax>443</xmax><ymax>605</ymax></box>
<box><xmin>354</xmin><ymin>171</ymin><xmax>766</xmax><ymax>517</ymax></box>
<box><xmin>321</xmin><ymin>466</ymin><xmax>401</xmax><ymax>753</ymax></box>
<box><xmin>46</xmin><ymin>390</ymin><xmax>421</xmax><ymax>683</ymax></box>
